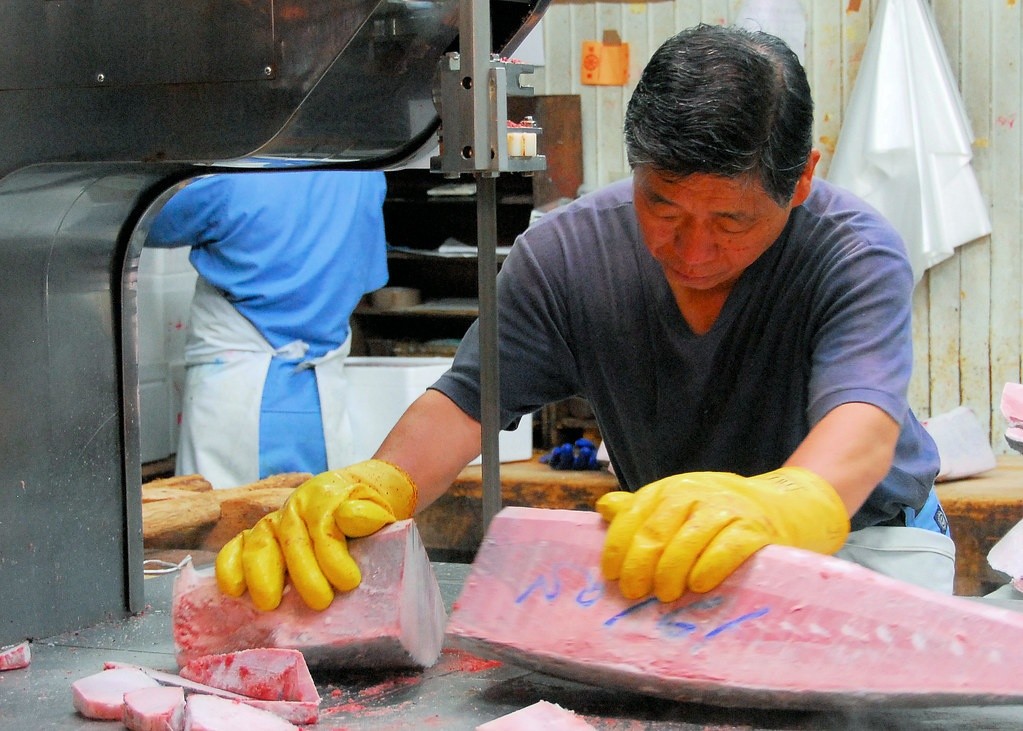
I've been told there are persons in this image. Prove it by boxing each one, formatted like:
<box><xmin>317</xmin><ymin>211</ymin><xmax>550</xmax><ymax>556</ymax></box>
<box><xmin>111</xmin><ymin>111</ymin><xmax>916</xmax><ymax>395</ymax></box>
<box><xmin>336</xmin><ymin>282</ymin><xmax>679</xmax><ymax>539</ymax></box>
<box><xmin>214</xmin><ymin>21</ymin><xmax>956</xmax><ymax>613</ymax></box>
<box><xmin>143</xmin><ymin>172</ymin><xmax>390</xmax><ymax>486</ymax></box>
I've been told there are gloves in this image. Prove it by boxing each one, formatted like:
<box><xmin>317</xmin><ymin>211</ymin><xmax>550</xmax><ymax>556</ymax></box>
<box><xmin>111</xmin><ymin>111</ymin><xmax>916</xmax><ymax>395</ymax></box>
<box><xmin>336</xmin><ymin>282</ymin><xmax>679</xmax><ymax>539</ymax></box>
<box><xmin>213</xmin><ymin>460</ymin><xmax>418</xmax><ymax>612</ymax></box>
<box><xmin>595</xmin><ymin>468</ymin><xmax>850</xmax><ymax>602</ymax></box>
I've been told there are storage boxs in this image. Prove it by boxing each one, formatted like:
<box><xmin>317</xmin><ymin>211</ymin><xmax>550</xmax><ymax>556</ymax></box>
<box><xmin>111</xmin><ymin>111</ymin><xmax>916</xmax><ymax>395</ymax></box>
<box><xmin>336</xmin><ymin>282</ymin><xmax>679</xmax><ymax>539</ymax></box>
<box><xmin>344</xmin><ymin>355</ymin><xmax>537</xmax><ymax>465</ymax></box>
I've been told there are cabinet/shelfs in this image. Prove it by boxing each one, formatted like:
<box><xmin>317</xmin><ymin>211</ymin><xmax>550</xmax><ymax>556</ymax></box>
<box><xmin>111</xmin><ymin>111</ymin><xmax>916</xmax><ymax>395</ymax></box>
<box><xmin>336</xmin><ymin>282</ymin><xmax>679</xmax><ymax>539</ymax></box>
<box><xmin>344</xmin><ymin>91</ymin><xmax>584</xmax><ymax>453</ymax></box>
